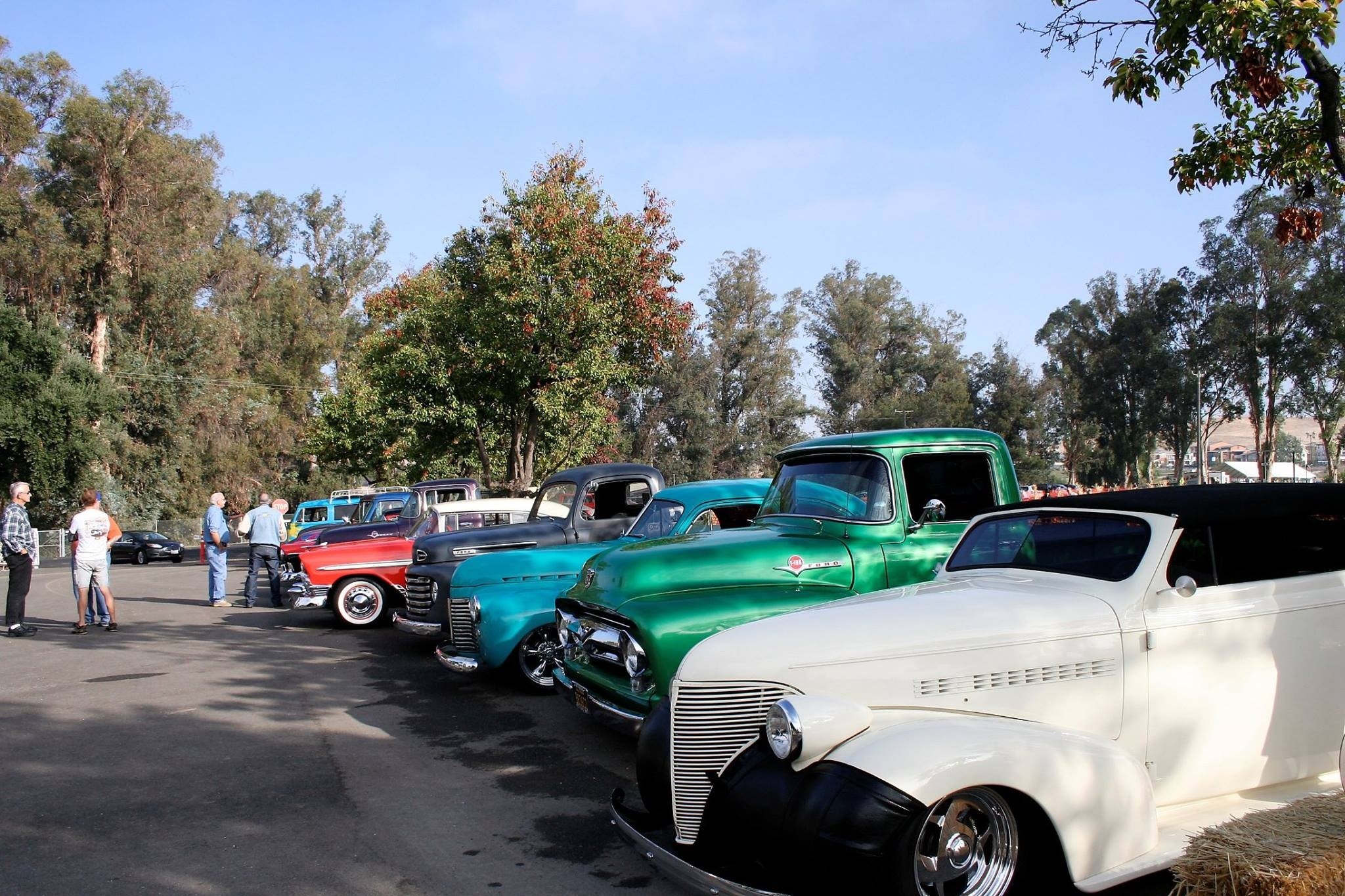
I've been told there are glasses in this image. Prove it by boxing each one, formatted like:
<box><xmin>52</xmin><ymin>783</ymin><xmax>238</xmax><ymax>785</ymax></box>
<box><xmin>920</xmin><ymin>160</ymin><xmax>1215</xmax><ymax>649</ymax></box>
<box><xmin>220</xmin><ymin>498</ymin><xmax>225</xmax><ymax>501</ymax></box>
<box><xmin>19</xmin><ymin>491</ymin><xmax>31</xmax><ymax>495</ymax></box>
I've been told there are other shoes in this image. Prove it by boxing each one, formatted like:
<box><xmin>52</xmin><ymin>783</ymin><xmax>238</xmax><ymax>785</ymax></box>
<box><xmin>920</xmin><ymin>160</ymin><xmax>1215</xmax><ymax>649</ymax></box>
<box><xmin>209</xmin><ymin>600</ymin><xmax>232</xmax><ymax>608</ymax></box>
<box><xmin>245</xmin><ymin>603</ymin><xmax>252</xmax><ymax>608</ymax></box>
<box><xmin>274</xmin><ymin>603</ymin><xmax>284</xmax><ymax>607</ymax></box>
<box><xmin>72</xmin><ymin>625</ymin><xmax>88</xmax><ymax>635</ymax></box>
<box><xmin>99</xmin><ymin>621</ymin><xmax>109</xmax><ymax>627</ymax></box>
<box><xmin>104</xmin><ymin>622</ymin><xmax>118</xmax><ymax>632</ymax></box>
<box><xmin>73</xmin><ymin>620</ymin><xmax>95</xmax><ymax>627</ymax></box>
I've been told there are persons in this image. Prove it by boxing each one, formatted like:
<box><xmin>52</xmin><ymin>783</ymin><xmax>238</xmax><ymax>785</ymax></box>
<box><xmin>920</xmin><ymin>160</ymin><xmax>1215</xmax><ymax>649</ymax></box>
<box><xmin>204</xmin><ymin>492</ymin><xmax>245</xmax><ymax>607</ymax></box>
<box><xmin>68</xmin><ymin>488</ymin><xmax>119</xmax><ymax>634</ymax></box>
<box><xmin>234</xmin><ymin>493</ymin><xmax>287</xmax><ymax>608</ymax></box>
<box><xmin>71</xmin><ymin>490</ymin><xmax>123</xmax><ymax>627</ymax></box>
<box><xmin>0</xmin><ymin>481</ymin><xmax>38</xmax><ymax>637</ymax></box>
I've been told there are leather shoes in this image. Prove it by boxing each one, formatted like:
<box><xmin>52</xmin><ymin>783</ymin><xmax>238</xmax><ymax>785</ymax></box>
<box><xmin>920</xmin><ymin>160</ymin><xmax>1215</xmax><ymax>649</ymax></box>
<box><xmin>8</xmin><ymin>625</ymin><xmax>39</xmax><ymax>637</ymax></box>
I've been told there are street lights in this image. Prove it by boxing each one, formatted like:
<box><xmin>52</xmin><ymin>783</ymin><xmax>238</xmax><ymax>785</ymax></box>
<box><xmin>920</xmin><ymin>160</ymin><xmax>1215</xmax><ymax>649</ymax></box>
<box><xmin>1306</xmin><ymin>432</ymin><xmax>1316</xmax><ymax>472</ymax></box>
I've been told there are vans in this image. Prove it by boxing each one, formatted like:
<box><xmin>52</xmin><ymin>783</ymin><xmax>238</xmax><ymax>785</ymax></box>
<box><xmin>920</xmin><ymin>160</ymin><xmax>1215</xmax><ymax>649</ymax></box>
<box><xmin>559</xmin><ymin>493</ymin><xmax>594</xmax><ymax>509</ymax></box>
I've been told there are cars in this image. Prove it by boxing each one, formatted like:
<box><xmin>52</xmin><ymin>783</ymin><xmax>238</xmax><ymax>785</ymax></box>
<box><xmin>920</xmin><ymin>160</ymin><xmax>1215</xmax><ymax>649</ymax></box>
<box><xmin>288</xmin><ymin>498</ymin><xmax>571</xmax><ymax>630</ymax></box>
<box><xmin>611</xmin><ymin>483</ymin><xmax>1344</xmax><ymax>895</ymax></box>
<box><xmin>432</xmin><ymin>478</ymin><xmax>868</xmax><ymax>697</ymax></box>
<box><xmin>110</xmin><ymin>530</ymin><xmax>185</xmax><ymax>565</ymax></box>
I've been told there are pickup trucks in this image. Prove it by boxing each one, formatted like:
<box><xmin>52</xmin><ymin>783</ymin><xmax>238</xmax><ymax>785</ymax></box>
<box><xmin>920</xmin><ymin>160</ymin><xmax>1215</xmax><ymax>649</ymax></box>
<box><xmin>552</xmin><ymin>427</ymin><xmax>1034</xmax><ymax>733</ymax></box>
<box><xmin>277</xmin><ymin>479</ymin><xmax>511</xmax><ymax>584</ymax></box>
<box><xmin>391</xmin><ymin>463</ymin><xmax>720</xmax><ymax>635</ymax></box>
